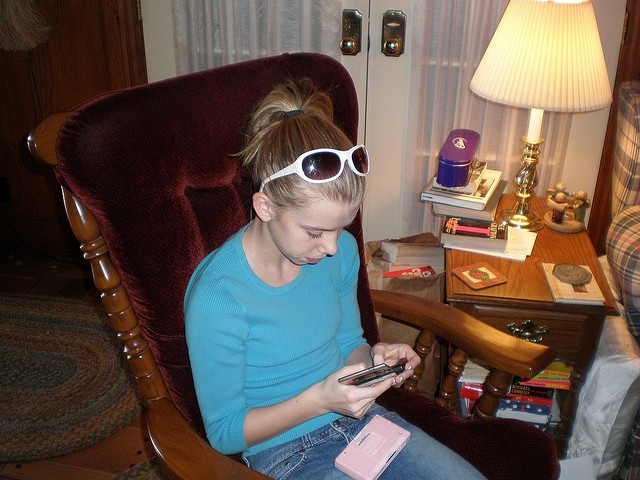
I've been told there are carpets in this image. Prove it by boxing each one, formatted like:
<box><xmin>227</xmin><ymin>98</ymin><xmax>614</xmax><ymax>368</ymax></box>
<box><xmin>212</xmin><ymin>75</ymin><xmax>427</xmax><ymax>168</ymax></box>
<box><xmin>2</xmin><ymin>296</ymin><xmax>141</xmax><ymax>457</ymax></box>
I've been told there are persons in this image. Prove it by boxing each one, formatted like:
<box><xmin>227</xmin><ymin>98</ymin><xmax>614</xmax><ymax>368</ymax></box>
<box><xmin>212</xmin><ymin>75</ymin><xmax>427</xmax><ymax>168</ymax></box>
<box><xmin>181</xmin><ymin>71</ymin><xmax>488</xmax><ymax>480</ymax></box>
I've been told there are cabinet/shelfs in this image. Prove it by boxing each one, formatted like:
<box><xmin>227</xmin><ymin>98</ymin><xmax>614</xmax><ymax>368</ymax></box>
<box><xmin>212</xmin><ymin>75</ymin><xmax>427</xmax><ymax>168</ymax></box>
<box><xmin>445</xmin><ymin>195</ymin><xmax>620</xmax><ymax>387</ymax></box>
<box><xmin>4</xmin><ymin>1</ymin><xmax>144</xmax><ymax>258</ymax></box>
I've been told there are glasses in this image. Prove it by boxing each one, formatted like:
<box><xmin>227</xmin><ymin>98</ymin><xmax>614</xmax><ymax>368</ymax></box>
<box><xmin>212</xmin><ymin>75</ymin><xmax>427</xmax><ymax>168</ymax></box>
<box><xmin>258</xmin><ymin>144</ymin><xmax>370</xmax><ymax>193</ymax></box>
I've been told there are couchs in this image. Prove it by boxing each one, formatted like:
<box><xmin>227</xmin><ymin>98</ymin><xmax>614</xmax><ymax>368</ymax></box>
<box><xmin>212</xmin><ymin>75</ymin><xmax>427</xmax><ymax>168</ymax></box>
<box><xmin>605</xmin><ymin>83</ymin><xmax>639</xmax><ymax>477</ymax></box>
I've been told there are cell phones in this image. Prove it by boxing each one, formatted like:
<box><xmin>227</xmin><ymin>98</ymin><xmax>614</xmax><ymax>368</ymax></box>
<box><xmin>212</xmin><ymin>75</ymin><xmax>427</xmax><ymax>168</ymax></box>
<box><xmin>337</xmin><ymin>357</ymin><xmax>408</xmax><ymax>388</ymax></box>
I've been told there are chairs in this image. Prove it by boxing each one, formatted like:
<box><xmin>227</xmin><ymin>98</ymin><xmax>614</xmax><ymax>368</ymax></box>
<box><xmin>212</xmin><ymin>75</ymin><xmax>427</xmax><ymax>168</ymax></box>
<box><xmin>26</xmin><ymin>52</ymin><xmax>552</xmax><ymax>479</ymax></box>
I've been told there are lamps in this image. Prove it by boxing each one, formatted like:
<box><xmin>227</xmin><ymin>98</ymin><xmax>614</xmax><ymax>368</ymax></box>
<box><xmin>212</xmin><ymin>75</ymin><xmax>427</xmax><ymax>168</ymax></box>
<box><xmin>470</xmin><ymin>2</ymin><xmax>613</xmax><ymax>231</ymax></box>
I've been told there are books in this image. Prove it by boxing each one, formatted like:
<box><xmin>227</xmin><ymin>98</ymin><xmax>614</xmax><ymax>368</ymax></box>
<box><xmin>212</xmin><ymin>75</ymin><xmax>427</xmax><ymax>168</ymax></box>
<box><xmin>440</xmin><ymin>215</ymin><xmax>509</xmax><ymax>251</ymax></box>
<box><xmin>421</xmin><ymin>159</ymin><xmax>509</xmax><ymax>221</ymax></box>
<box><xmin>542</xmin><ymin>262</ymin><xmax>605</xmax><ymax>306</ymax></box>
<box><xmin>461</xmin><ymin>361</ymin><xmax>574</xmax><ymax>440</ymax></box>
